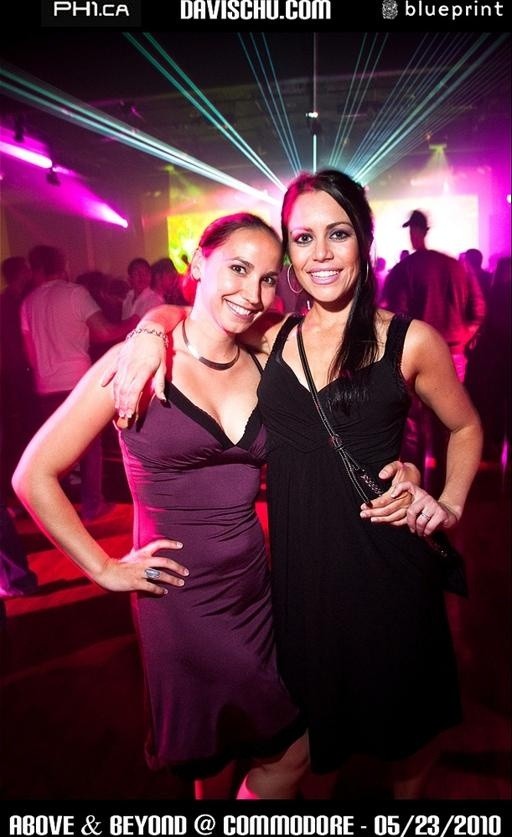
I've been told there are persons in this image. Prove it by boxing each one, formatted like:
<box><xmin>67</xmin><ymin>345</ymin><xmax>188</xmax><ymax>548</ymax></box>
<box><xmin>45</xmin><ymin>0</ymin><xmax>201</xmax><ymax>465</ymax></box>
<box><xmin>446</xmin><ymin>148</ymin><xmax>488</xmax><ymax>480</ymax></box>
<box><xmin>102</xmin><ymin>168</ymin><xmax>483</xmax><ymax>801</ymax></box>
<box><xmin>0</xmin><ymin>244</ymin><xmax>510</xmax><ymax>686</ymax></box>
<box><xmin>13</xmin><ymin>212</ymin><xmax>422</xmax><ymax>798</ymax></box>
<box><xmin>379</xmin><ymin>207</ymin><xmax>484</xmax><ymax>474</ymax></box>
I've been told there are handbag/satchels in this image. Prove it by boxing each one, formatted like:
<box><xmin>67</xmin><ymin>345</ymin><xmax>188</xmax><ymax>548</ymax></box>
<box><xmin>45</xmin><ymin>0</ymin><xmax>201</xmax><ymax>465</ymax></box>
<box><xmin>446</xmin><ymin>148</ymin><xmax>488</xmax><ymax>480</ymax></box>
<box><xmin>432</xmin><ymin>543</ymin><xmax>468</xmax><ymax>596</ymax></box>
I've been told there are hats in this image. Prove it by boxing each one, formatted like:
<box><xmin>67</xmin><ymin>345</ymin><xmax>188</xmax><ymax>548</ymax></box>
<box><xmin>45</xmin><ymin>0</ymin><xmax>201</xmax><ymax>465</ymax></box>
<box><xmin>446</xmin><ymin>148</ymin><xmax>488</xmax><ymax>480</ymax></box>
<box><xmin>403</xmin><ymin>211</ymin><xmax>426</xmax><ymax>228</ymax></box>
